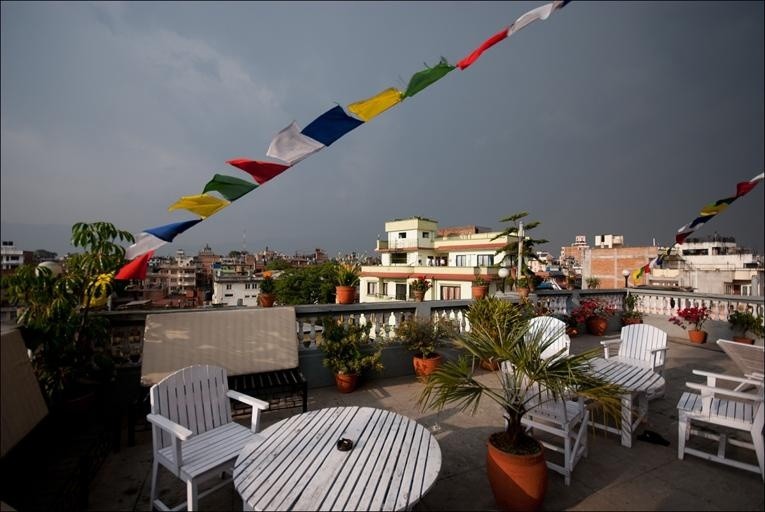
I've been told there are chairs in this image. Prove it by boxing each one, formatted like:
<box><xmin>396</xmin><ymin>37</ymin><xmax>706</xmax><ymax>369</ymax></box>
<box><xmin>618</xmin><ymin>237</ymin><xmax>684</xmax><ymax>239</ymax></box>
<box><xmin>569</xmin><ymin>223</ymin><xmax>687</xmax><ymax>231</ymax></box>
<box><xmin>146</xmin><ymin>362</ymin><xmax>270</xmax><ymax>510</ymax></box>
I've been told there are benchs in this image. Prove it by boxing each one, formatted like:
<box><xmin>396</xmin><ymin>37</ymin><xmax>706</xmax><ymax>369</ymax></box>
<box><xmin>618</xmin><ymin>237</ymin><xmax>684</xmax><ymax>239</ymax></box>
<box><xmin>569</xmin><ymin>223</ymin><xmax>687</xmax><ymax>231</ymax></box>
<box><xmin>140</xmin><ymin>306</ymin><xmax>307</xmax><ymax>413</ymax></box>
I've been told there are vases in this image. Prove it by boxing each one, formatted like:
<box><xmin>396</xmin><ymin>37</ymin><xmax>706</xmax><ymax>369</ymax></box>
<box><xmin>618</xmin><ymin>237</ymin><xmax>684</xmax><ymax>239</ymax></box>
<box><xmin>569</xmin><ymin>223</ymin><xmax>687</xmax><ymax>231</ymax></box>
<box><xmin>586</xmin><ymin>319</ymin><xmax>708</xmax><ymax>344</ymax></box>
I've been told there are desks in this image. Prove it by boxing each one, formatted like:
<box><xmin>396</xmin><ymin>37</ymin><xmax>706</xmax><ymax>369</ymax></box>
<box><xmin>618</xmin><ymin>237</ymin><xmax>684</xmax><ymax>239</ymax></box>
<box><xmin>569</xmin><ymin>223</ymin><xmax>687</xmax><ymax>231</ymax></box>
<box><xmin>232</xmin><ymin>405</ymin><xmax>442</xmax><ymax>511</ymax></box>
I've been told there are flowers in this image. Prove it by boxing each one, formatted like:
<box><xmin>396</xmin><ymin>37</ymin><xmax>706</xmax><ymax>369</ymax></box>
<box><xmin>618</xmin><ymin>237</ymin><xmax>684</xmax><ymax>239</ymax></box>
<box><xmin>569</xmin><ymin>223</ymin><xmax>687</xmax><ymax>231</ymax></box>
<box><xmin>571</xmin><ymin>298</ymin><xmax>710</xmax><ymax>329</ymax></box>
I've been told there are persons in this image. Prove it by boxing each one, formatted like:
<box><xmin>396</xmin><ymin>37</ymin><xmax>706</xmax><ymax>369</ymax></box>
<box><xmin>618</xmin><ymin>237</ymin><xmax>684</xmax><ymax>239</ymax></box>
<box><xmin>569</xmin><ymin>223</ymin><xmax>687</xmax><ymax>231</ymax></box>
<box><xmin>418</xmin><ymin>256</ymin><xmax>447</xmax><ymax>267</ymax></box>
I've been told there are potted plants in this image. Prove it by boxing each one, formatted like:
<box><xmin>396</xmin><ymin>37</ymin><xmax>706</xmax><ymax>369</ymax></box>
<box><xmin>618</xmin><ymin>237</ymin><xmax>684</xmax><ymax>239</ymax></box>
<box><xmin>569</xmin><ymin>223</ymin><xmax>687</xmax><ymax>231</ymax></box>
<box><xmin>414</xmin><ymin>292</ymin><xmax>664</xmax><ymax>511</ymax></box>
<box><xmin>727</xmin><ymin>305</ymin><xmax>764</xmax><ymax>345</ymax></box>
<box><xmin>257</xmin><ymin>261</ymin><xmax>530</xmax><ymax>392</ymax></box>
